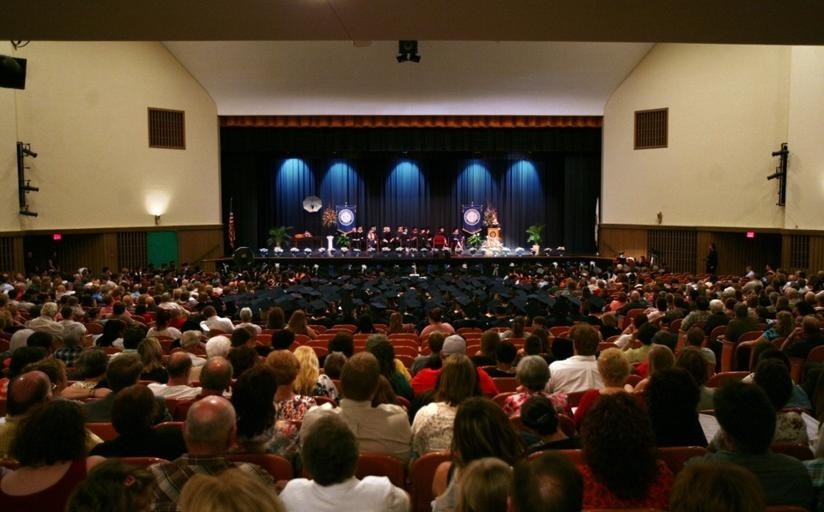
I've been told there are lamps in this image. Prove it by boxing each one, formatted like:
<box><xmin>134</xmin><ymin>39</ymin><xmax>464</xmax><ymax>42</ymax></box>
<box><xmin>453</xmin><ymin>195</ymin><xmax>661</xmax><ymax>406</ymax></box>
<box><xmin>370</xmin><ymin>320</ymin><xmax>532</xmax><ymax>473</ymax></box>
<box><xmin>763</xmin><ymin>140</ymin><xmax>789</xmax><ymax>210</ymax></box>
<box><xmin>15</xmin><ymin>138</ymin><xmax>43</xmax><ymax>222</ymax></box>
<box><xmin>152</xmin><ymin>215</ymin><xmax>161</xmax><ymax>225</ymax></box>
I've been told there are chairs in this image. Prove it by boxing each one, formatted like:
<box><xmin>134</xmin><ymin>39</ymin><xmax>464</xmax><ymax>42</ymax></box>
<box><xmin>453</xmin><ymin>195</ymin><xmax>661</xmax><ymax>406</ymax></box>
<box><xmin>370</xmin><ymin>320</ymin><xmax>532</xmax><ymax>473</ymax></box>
<box><xmin>345</xmin><ymin>233</ymin><xmax>465</xmax><ymax>252</ymax></box>
<box><xmin>0</xmin><ymin>266</ymin><xmax>823</xmax><ymax>512</ymax></box>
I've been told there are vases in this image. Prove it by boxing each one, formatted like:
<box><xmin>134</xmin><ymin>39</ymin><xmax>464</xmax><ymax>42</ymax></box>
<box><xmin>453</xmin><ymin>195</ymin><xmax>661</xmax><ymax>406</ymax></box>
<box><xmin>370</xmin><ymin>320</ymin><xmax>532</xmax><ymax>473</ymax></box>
<box><xmin>326</xmin><ymin>235</ymin><xmax>333</xmax><ymax>249</ymax></box>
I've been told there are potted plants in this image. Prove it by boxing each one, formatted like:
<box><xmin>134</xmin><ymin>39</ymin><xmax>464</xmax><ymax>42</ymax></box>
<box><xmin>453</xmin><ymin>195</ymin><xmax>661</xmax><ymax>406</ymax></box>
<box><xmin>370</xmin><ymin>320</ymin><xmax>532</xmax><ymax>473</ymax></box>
<box><xmin>525</xmin><ymin>222</ymin><xmax>545</xmax><ymax>256</ymax></box>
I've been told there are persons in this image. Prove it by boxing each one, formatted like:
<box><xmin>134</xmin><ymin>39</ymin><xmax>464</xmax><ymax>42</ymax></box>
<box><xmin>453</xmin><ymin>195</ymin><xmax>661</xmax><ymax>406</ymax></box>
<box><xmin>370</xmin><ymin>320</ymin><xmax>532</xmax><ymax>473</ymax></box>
<box><xmin>0</xmin><ymin>224</ymin><xmax>823</xmax><ymax>510</ymax></box>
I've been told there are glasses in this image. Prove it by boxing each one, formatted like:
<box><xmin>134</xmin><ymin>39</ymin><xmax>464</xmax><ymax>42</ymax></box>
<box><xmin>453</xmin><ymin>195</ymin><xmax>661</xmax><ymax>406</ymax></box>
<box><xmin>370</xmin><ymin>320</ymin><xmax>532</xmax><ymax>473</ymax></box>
<box><xmin>49</xmin><ymin>382</ymin><xmax>57</xmax><ymax>392</ymax></box>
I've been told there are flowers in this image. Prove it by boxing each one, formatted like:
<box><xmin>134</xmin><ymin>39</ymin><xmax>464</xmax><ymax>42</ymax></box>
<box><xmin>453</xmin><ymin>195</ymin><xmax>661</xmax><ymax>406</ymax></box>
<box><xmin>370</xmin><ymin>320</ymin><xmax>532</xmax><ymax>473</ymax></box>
<box><xmin>481</xmin><ymin>203</ymin><xmax>499</xmax><ymax>227</ymax></box>
<box><xmin>321</xmin><ymin>206</ymin><xmax>337</xmax><ymax>235</ymax></box>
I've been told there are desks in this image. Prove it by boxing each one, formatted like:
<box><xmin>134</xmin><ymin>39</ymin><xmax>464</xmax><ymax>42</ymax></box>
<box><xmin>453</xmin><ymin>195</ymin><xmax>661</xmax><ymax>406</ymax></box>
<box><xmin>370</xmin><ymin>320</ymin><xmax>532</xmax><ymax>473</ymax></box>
<box><xmin>294</xmin><ymin>235</ymin><xmax>323</xmax><ymax>249</ymax></box>
